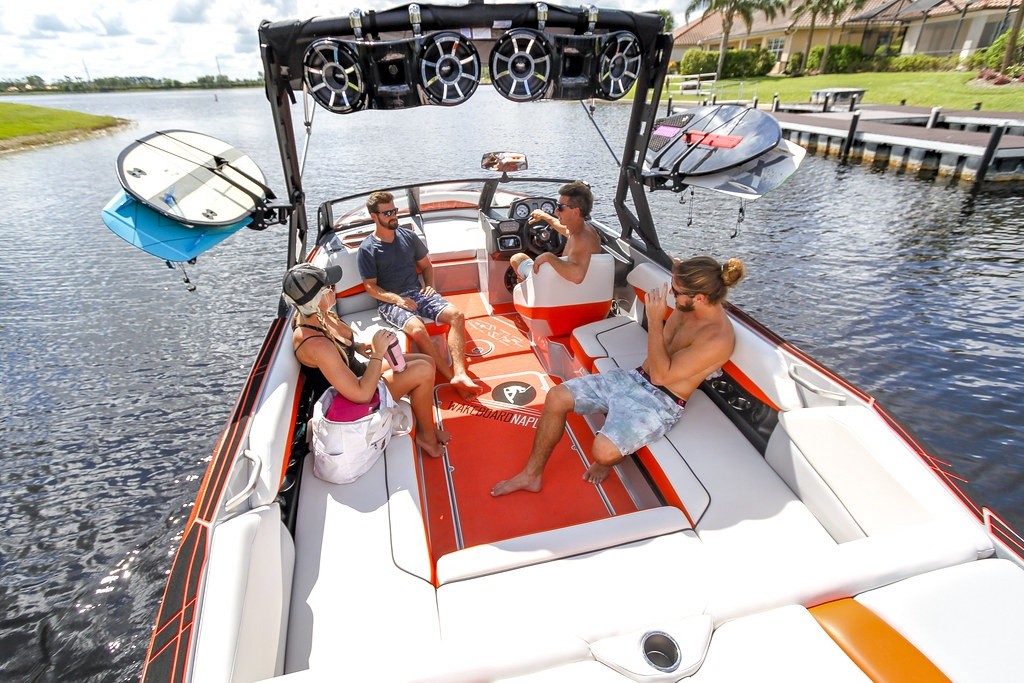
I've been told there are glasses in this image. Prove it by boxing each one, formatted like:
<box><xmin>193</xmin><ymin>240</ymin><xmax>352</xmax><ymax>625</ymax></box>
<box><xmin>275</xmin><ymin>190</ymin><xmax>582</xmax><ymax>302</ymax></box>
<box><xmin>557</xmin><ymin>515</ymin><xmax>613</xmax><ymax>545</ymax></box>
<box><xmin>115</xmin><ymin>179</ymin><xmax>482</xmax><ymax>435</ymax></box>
<box><xmin>555</xmin><ymin>201</ymin><xmax>572</xmax><ymax>212</ymax></box>
<box><xmin>372</xmin><ymin>207</ymin><xmax>399</xmax><ymax>216</ymax></box>
<box><xmin>669</xmin><ymin>283</ymin><xmax>690</xmax><ymax>299</ymax></box>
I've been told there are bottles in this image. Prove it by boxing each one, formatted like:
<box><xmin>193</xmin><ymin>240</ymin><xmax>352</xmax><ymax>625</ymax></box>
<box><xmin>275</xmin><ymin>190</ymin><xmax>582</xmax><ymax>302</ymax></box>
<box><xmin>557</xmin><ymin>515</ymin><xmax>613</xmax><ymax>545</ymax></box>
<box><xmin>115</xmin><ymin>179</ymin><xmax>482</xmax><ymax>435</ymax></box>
<box><xmin>382</xmin><ymin>336</ymin><xmax>407</xmax><ymax>374</ymax></box>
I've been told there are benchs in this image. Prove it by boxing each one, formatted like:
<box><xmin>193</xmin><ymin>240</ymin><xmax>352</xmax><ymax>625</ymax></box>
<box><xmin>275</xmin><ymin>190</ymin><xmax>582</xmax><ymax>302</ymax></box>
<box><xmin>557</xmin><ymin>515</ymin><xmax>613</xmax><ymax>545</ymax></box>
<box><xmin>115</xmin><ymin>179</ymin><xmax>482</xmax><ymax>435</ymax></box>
<box><xmin>248</xmin><ymin>249</ymin><xmax>435</xmax><ymax>675</ymax></box>
<box><xmin>568</xmin><ymin>262</ymin><xmax>804</xmax><ymax>529</ymax></box>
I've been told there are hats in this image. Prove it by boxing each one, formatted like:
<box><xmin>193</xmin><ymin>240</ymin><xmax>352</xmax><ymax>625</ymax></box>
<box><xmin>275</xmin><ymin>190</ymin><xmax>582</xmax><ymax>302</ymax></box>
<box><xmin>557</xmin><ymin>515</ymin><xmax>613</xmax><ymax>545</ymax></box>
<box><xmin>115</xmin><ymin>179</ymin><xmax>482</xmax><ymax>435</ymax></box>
<box><xmin>282</xmin><ymin>262</ymin><xmax>343</xmax><ymax>305</ymax></box>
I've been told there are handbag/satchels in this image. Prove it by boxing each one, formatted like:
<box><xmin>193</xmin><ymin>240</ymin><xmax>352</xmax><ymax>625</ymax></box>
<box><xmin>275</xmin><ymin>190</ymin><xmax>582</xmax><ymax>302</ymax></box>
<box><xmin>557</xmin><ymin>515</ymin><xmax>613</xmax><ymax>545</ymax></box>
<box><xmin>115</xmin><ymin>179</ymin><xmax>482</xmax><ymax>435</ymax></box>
<box><xmin>307</xmin><ymin>377</ymin><xmax>415</xmax><ymax>486</ymax></box>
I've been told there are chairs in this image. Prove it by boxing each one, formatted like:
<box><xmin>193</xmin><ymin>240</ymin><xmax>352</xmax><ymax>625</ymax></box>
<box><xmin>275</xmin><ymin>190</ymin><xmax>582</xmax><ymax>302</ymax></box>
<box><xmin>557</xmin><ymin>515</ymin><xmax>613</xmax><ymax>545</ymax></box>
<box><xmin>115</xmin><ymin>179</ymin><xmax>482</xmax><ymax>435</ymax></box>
<box><xmin>512</xmin><ymin>254</ymin><xmax>614</xmax><ymax>350</ymax></box>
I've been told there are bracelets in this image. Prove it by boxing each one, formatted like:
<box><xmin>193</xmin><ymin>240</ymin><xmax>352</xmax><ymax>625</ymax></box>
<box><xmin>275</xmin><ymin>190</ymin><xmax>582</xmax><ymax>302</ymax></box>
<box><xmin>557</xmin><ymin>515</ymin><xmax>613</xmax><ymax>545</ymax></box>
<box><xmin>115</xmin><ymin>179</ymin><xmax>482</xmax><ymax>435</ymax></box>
<box><xmin>368</xmin><ymin>356</ymin><xmax>382</xmax><ymax>361</ymax></box>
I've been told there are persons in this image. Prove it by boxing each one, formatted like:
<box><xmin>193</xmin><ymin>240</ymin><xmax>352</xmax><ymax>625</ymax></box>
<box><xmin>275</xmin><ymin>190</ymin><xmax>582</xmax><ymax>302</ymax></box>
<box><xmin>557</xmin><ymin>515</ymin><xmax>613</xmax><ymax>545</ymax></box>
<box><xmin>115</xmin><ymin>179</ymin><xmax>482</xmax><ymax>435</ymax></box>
<box><xmin>489</xmin><ymin>257</ymin><xmax>743</xmax><ymax>497</ymax></box>
<box><xmin>509</xmin><ymin>182</ymin><xmax>600</xmax><ymax>285</ymax></box>
<box><xmin>356</xmin><ymin>192</ymin><xmax>483</xmax><ymax>403</ymax></box>
<box><xmin>282</xmin><ymin>263</ymin><xmax>453</xmax><ymax>457</ymax></box>
